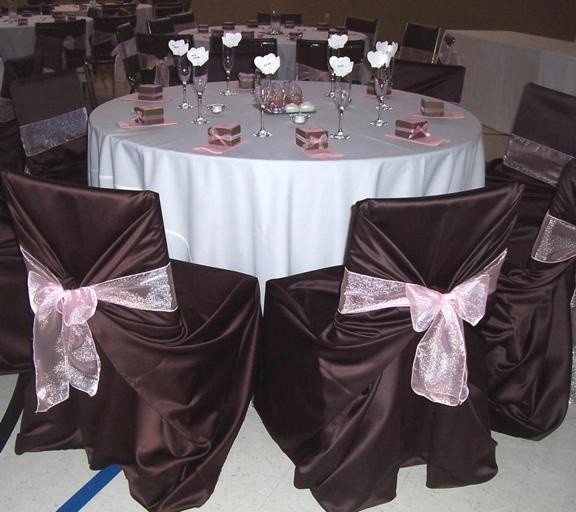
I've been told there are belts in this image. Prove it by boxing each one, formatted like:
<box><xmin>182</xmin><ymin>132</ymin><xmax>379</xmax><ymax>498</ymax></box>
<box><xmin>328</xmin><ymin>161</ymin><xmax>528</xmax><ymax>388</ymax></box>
<box><xmin>331</xmin><ymin>74</ymin><xmax>351</xmax><ymax>142</ymax></box>
<box><xmin>191</xmin><ymin>64</ymin><xmax>209</xmax><ymax>127</ymax></box>
<box><xmin>177</xmin><ymin>54</ymin><xmax>193</xmax><ymax>112</ymax></box>
<box><xmin>324</xmin><ymin>47</ymin><xmax>343</xmax><ymax>97</ymax></box>
<box><xmin>253</xmin><ymin>69</ymin><xmax>271</xmax><ymax>139</ymax></box>
<box><xmin>368</xmin><ymin>59</ymin><xmax>394</xmax><ymax>128</ymax></box>
<box><xmin>221</xmin><ymin>42</ymin><xmax>236</xmax><ymax>97</ymax></box>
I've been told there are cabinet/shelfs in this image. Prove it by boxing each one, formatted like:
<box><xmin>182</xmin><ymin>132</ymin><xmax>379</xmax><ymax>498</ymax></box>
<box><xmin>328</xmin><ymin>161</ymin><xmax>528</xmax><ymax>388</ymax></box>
<box><xmin>262</xmin><ymin>80</ymin><xmax>314</xmax><ymax>124</ymax></box>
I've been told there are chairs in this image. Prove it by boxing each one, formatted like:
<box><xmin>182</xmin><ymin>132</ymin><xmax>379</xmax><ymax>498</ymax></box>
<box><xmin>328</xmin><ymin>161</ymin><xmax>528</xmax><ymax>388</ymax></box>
<box><xmin>467</xmin><ymin>158</ymin><xmax>573</xmax><ymax>442</ymax></box>
<box><xmin>250</xmin><ymin>182</ymin><xmax>526</xmax><ymax>510</ymax></box>
<box><xmin>0</xmin><ymin>1</ymin><xmax>576</xmax><ymax>372</ymax></box>
<box><xmin>2</xmin><ymin>169</ymin><xmax>264</xmax><ymax>510</ymax></box>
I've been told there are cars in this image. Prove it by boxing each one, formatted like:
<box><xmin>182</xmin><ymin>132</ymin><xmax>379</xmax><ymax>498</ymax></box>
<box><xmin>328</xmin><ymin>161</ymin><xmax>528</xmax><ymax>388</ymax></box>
<box><xmin>268</xmin><ymin>8</ymin><xmax>282</xmax><ymax>34</ymax></box>
<box><xmin>6</xmin><ymin>3</ymin><xmax>17</xmax><ymax>22</ymax></box>
<box><xmin>294</xmin><ymin>24</ymin><xmax>304</xmax><ymax>37</ymax></box>
